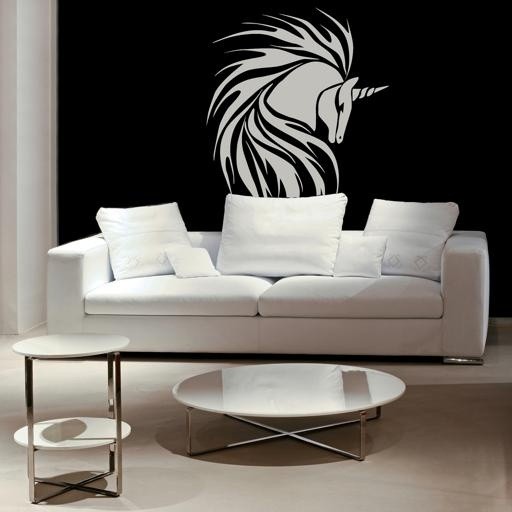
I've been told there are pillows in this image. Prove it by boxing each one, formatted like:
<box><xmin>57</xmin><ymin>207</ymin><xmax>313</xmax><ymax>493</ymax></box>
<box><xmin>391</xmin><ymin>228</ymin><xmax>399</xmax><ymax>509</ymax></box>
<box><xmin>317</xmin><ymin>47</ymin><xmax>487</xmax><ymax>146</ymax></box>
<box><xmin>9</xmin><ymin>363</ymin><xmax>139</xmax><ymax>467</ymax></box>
<box><xmin>164</xmin><ymin>244</ymin><xmax>220</xmax><ymax>278</ymax></box>
<box><xmin>333</xmin><ymin>237</ymin><xmax>389</xmax><ymax>276</ymax></box>
<box><xmin>218</xmin><ymin>192</ymin><xmax>349</xmax><ymax>276</ymax></box>
<box><xmin>96</xmin><ymin>201</ymin><xmax>192</xmax><ymax>279</ymax></box>
<box><xmin>357</xmin><ymin>197</ymin><xmax>459</xmax><ymax>281</ymax></box>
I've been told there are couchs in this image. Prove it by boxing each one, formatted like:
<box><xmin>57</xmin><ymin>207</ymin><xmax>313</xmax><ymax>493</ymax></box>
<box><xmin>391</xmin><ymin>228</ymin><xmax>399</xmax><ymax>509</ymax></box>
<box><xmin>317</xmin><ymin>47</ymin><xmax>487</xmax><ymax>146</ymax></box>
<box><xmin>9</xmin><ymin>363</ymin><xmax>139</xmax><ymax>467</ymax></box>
<box><xmin>46</xmin><ymin>227</ymin><xmax>489</xmax><ymax>367</ymax></box>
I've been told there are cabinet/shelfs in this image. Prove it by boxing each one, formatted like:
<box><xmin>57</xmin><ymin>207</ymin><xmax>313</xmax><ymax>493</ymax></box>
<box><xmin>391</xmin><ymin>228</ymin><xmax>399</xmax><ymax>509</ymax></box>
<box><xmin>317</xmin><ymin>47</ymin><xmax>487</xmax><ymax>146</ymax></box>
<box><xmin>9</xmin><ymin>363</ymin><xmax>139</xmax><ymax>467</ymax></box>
<box><xmin>13</xmin><ymin>337</ymin><xmax>132</xmax><ymax>505</ymax></box>
